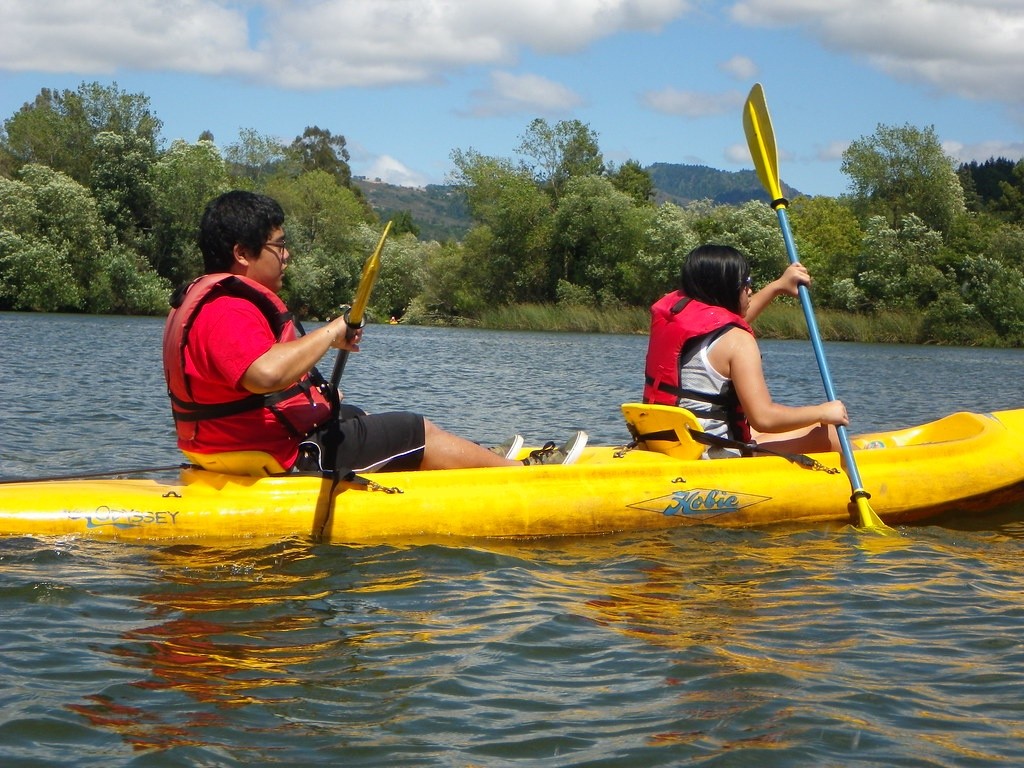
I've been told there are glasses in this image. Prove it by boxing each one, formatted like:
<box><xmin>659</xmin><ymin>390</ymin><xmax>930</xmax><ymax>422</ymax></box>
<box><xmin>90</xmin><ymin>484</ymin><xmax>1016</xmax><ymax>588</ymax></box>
<box><xmin>238</xmin><ymin>240</ymin><xmax>287</xmax><ymax>252</ymax></box>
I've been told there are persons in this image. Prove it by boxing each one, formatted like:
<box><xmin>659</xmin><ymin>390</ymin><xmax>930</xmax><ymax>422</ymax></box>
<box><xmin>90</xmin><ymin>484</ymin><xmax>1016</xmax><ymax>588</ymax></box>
<box><xmin>161</xmin><ymin>190</ymin><xmax>588</xmax><ymax>472</ymax></box>
<box><xmin>643</xmin><ymin>244</ymin><xmax>861</xmax><ymax>459</ymax></box>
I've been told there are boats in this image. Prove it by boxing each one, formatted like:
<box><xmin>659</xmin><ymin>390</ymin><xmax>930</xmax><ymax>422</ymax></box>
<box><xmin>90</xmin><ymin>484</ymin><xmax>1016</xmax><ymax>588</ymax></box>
<box><xmin>0</xmin><ymin>405</ymin><xmax>1019</xmax><ymax>556</ymax></box>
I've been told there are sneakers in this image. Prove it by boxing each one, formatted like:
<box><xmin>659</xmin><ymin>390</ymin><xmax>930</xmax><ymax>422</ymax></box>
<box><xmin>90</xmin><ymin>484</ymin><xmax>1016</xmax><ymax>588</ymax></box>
<box><xmin>489</xmin><ymin>435</ymin><xmax>524</xmax><ymax>460</ymax></box>
<box><xmin>526</xmin><ymin>431</ymin><xmax>587</xmax><ymax>465</ymax></box>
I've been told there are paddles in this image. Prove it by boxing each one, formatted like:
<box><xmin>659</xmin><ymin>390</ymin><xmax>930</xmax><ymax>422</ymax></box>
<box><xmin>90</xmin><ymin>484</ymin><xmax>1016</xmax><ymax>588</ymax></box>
<box><xmin>742</xmin><ymin>82</ymin><xmax>884</xmax><ymax>529</ymax></box>
<box><xmin>329</xmin><ymin>220</ymin><xmax>389</xmax><ymax>388</ymax></box>
